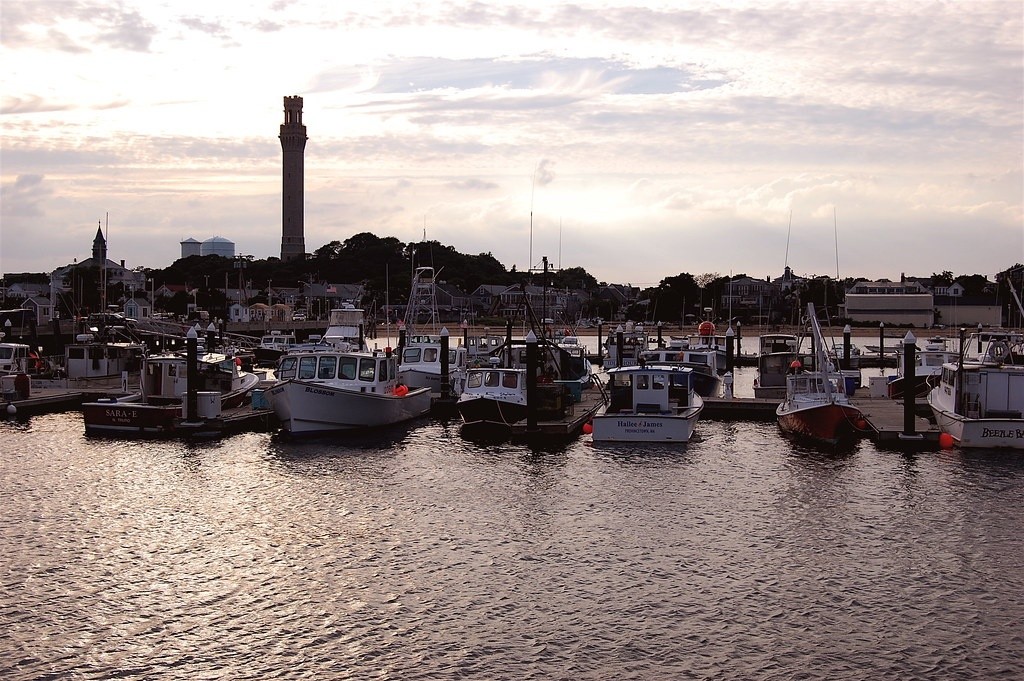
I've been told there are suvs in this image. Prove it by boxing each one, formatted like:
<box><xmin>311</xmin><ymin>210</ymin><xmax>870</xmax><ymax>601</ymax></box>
<box><xmin>87</xmin><ymin>313</ymin><xmax>138</xmax><ymax>328</ymax></box>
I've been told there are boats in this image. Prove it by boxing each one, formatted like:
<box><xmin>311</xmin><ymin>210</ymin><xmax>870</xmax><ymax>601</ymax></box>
<box><xmin>261</xmin><ymin>262</ymin><xmax>433</xmax><ymax>436</ymax></box>
<box><xmin>591</xmin><ymin>362</ymin><xmax>704</xmax><ymax>443</ymax></box>
<box><xmin>601</xmin><ymin>322</ymin><xmax>976</xmax><ymax>401</ymax></box>
<box><xmin>457</xmin><ymin>364</ymin><xmax>528</xmax><ymax>433</ymax></box>
<box><xmin>773</xmin><ymin>304</ymin><xmax>866</xmax><ymax>450</ymax></box>
<box><xmin>0</xmin><ymin>306</ymin><xmax>150</xmax><ymax>386</ymax></box>
<box><xmin>249</xmin><ymin>257</ymin><xmax>595</xmax><ymax>393</ymax></box>
<box><xmin>927</xmin><ymin>331</ymin><xmax>1024</xmax><ymax>449</ymax></box>
<box><xmin>80</xmin><ymin>323</ymin><xmax>260</xmax><ymax>435</ymax></box>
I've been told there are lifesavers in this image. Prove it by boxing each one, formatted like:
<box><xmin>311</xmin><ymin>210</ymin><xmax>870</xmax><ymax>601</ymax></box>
<box><xmin>554</xmin><ymin>328</ymin><xmax>571</xmax><ymax>336</ymax></box>
<box><xmin>989</xmin><ymin>341</ymin><xmax>1008</xmax><ymax>360</ymax></box>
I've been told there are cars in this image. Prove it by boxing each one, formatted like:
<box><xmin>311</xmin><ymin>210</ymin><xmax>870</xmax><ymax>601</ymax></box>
<box><xmin>540</xmin><ymin>316</ymin><xmax>553</xmax><ymax>324</ymax></box>
<box><xmin>293</xmin><ymin>313</ymin><xmax>306</xmax><ymax>321</ymax></box>
<box><xmin>153</xmin><ymin>312</ymin><xmax>175</xmax><ymax>321</ymax></box>
